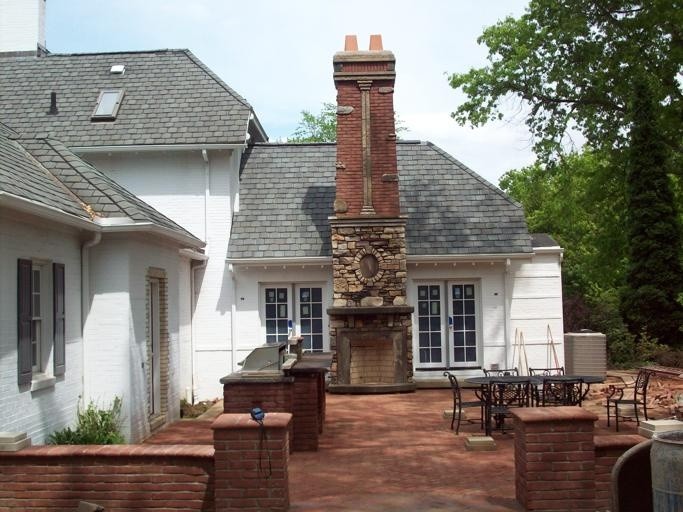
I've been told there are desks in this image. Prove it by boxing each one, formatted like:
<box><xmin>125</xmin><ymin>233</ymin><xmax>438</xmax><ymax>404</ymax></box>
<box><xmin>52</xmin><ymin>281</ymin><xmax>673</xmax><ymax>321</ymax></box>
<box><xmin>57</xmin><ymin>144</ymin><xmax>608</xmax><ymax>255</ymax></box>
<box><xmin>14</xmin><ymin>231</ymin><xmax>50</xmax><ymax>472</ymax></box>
<box><xmin>464</xmin><ymin>375</ymin><xmax>607</xmax><ymax>436</ymax></box>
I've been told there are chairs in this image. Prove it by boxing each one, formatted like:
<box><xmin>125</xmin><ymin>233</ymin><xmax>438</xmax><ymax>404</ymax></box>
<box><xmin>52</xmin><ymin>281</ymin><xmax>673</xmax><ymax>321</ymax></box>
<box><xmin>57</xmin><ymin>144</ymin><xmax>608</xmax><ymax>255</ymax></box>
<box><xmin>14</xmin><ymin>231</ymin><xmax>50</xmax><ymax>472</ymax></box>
<box><xmin>485</xmin><ymin>379</ymin><xmax>531</xmax><ymax>436</ymax></box>
<box><xmin>483</xmin><ymin>367</ymin><xmax>522</xmax><ymax>433</ymax></box>
<box><xmin>542</xmin><ymin>378</ymin><xmax>581</xmax><ymax>407</ymax></box>
<box><xmin>607</xmin><ymin>370</ymin><xmax>652</xmax><ymax>432</ymax></box>
<box><xmin>529</xmin><ymin>367</ymin><xmax>566</xmax><ymax>406</ymax></box>
<box><xmin>443</xmin><ymin>371</ymin><xmax>489</xmax><ymax>435</ymax></box>
